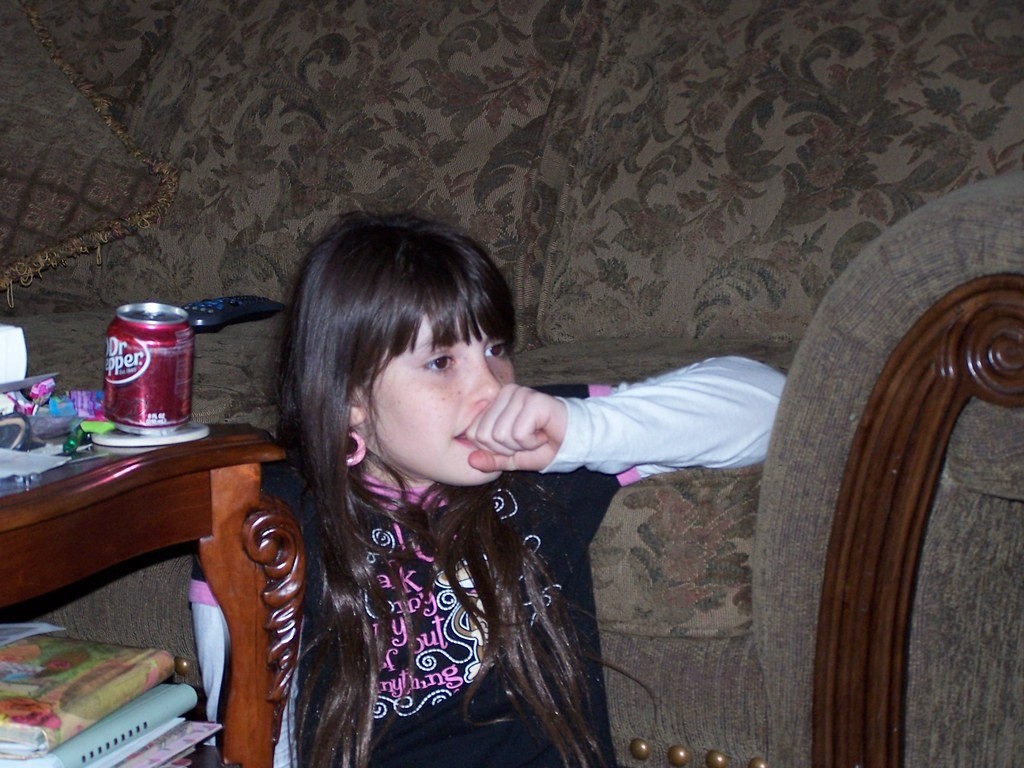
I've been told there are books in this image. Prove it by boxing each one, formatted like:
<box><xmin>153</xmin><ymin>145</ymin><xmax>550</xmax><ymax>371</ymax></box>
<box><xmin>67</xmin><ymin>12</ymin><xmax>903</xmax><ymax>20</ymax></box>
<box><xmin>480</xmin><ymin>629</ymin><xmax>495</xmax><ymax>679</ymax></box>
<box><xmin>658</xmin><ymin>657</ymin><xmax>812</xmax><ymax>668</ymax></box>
<box><xmin>1</xmin><ymin>633</ymin><xmax>222</xmax><ymax>768</ymax></box>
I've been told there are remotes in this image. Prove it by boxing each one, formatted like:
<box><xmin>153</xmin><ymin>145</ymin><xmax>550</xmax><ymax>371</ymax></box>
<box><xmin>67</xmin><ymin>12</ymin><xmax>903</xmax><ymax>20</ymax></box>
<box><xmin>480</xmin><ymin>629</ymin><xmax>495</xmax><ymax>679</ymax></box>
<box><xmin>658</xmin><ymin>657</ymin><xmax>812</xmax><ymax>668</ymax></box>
<box><xmin>182</xmin><ymin>296</ymin><xmax>286</xmax><ymax>333</ymax></box>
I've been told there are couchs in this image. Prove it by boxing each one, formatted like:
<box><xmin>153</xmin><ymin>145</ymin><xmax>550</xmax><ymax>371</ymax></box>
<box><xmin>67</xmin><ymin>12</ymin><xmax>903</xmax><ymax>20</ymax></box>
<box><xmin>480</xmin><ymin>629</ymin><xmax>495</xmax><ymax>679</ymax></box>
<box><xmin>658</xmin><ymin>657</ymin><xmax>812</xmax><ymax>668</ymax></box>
<box><xmin>0</xmin><ymin>0</ymin><xmax>1024</xmax><ymax>768</ymax></box>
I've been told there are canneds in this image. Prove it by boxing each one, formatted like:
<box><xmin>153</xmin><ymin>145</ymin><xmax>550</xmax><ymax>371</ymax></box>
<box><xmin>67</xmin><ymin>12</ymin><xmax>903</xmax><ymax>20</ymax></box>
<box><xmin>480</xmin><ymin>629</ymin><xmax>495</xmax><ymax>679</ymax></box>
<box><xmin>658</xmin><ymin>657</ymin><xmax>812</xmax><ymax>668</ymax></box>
<box><xmin>102</xmin><ymin>302</ymin><xmax>194</xmax><ymax>436</ymax></box>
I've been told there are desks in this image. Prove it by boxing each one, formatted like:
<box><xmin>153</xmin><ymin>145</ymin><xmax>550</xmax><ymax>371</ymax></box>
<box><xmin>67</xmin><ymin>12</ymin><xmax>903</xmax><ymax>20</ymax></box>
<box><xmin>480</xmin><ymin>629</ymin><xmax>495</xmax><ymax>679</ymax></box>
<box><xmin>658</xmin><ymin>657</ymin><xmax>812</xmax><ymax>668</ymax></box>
<box><xmin>0</xmin><ymin>421</ymin><xmax>309</xmax><ymax>768</ymax></box>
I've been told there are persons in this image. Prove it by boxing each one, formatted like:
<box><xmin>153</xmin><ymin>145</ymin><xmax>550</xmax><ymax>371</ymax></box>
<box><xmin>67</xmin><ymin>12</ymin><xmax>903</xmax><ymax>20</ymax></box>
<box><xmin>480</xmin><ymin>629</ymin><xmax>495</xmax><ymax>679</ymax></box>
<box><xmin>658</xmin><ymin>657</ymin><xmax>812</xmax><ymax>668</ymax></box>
<box><xmin>255</xmin><ymin>206</ymin><xmax>787</xmax><ymax>767</ymax></box>
